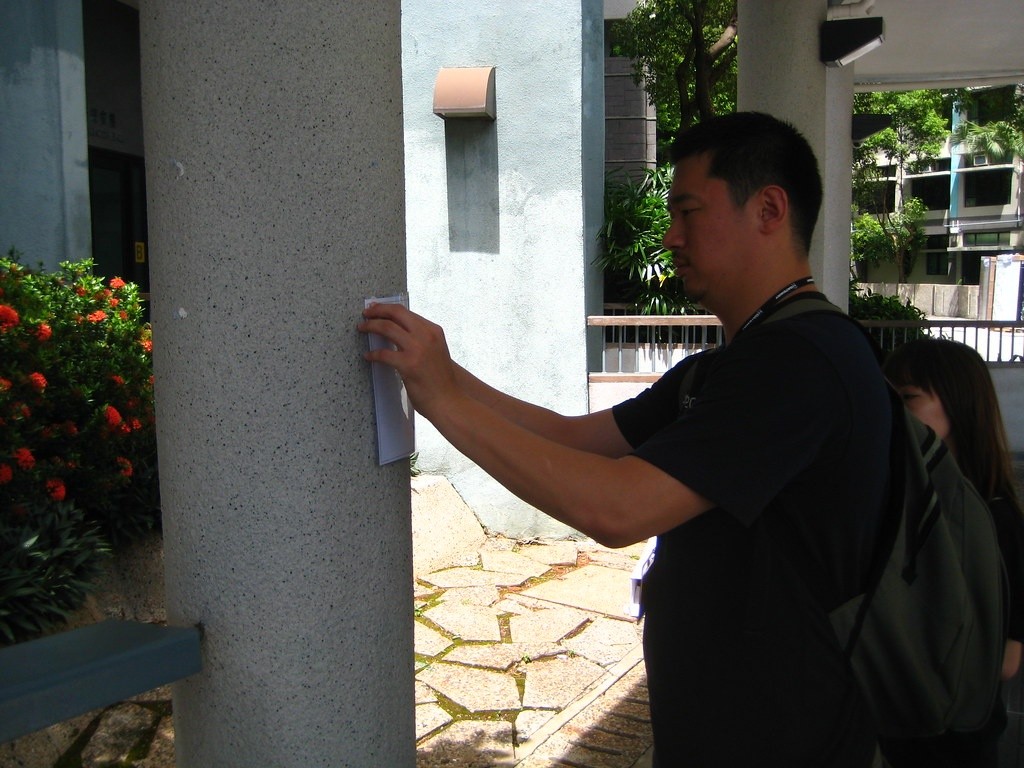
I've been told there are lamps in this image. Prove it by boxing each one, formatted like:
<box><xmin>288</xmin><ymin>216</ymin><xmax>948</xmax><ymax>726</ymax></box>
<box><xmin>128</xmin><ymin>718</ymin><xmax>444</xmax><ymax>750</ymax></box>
<box><xmin>820</xmin><ymin>15</ymin><xmax>887</xmax><ymax>68</ymax></box>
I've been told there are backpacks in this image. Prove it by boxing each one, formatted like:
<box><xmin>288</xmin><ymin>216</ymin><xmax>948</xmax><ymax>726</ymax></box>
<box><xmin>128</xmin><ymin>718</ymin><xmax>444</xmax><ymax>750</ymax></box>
<box><xmin>754</xmin><ymin>298</ymin><xmax>1011</xmax><ymax>739</ymax></box>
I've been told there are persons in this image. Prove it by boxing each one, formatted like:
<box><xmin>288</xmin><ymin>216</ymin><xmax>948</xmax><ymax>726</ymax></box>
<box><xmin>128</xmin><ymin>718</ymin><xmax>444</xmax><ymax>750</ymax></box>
<box><xmin>880</xmin><ymin>338</ymin><xmax>1024</xmax><ymax>768</ymax></box>
<box><xmin>354</xmin><ymin>111</ymin><xmax>905</xmax><ymax>768</ymax></box>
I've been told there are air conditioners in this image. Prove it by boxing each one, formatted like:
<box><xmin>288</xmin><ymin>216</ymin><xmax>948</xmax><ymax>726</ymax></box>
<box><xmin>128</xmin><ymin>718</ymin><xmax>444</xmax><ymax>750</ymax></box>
<box><xmin>918</xmin><ymin>161</ymin><xmax>940</xmax><ymax>174</ymax></box>
<box><xmin>974</xmin><ymin>154</ymin><xmax>996</xmax><ymax>166</ymax></box>
